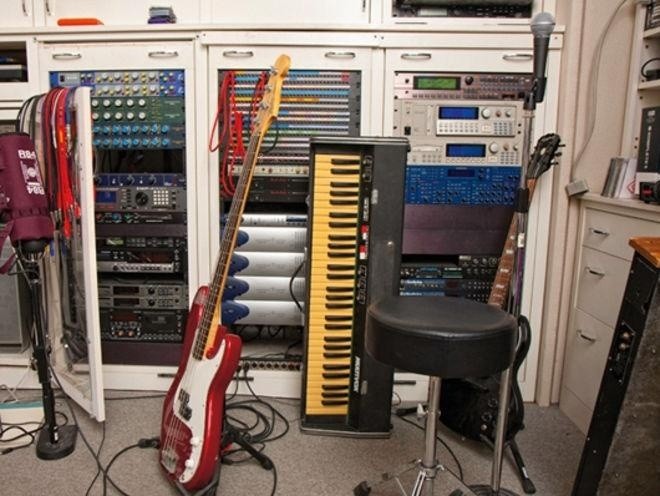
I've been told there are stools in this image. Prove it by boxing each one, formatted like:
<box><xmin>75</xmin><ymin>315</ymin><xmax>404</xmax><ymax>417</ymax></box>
<box><xmin>354</xmin><ymin>295</ymin><xmax>518</xmax><ymax>496</ymax></box>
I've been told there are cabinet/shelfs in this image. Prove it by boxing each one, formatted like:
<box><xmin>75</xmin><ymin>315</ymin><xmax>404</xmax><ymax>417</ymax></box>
<box><xmin>558</xmin><ymin>193</ymin><xmax>660</xmax><ymax>437</ymax></box>
<box><xmin>619</xmin><ymin>0</ymin><xmax>660</xmax><ymax>199</ymax></box>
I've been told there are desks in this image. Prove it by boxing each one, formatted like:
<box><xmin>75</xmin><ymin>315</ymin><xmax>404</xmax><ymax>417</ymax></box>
<box><xmin>571</xmin><ymin>236</ymin><xmax>660</xmax><ymax>496</ymax></box>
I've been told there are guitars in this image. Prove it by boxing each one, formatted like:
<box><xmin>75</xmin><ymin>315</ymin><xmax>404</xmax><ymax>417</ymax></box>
<box><xmin>439</xmin><ymin>133</ymin><xmax>566</xmax><ymax>442</ymax></box>
<box><xmin>157</xmin><ymin>55</ymin><xmax>291</xmax><ymax>496</ymax></box>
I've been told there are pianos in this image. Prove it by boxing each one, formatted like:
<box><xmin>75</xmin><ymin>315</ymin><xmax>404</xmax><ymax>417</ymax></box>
<box><xmin>298</xmin><ymin>134</ymin><xmax>411</xmax><ymax>439</ymax></box>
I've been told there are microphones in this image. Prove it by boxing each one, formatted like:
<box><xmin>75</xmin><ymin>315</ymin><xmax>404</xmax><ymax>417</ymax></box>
<box><xmin>531</xmin><ymin>11</ymin><xmax>556</xmax><ymax>103</ymax></box>
